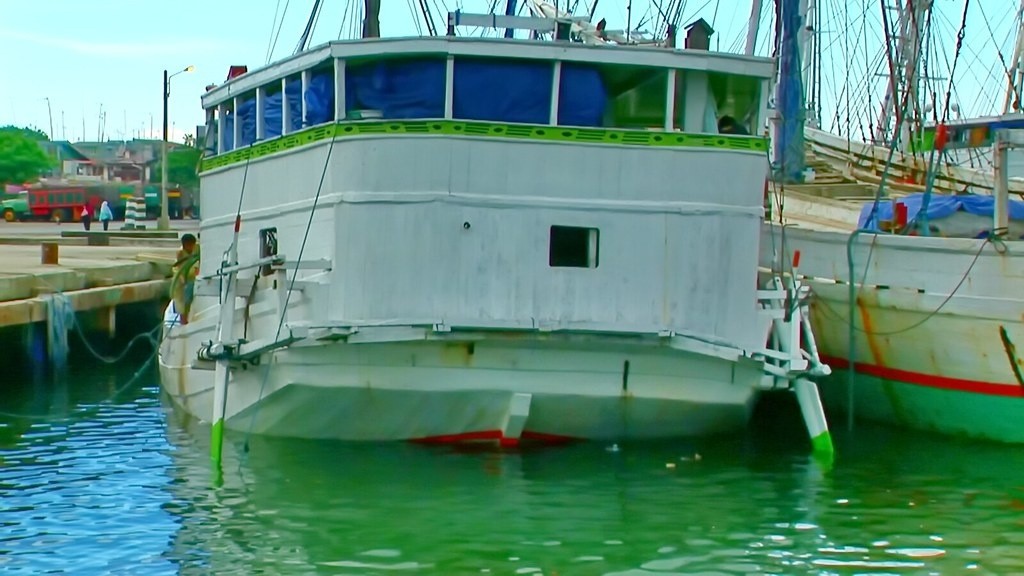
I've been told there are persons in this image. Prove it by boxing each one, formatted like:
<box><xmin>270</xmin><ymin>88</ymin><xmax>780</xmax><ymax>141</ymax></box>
<box><xmin>98</xmin><ymin>201</ymin><xmax>113</xmax><ymax>231</ymax></box>
<box><xmin>81</xmin><ymin>206</ymin><xmax>91</xmax><ymax>230</ymax></box>
<box><xmin>172</xmin><ymin>233</ymin><xmax>200</xmax><ymax>325</ymax></box>
<box><xmin>710</xmin><ymin>74</ymin><xmax>751</xmax><ymax>135</ymax></box>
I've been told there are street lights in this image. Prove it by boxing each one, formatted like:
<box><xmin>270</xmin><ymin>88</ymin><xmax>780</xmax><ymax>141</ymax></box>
<box><xmin>161</xmin><ymin>65</ymin><xmax>195</xmax><ymax>230</ymax></box>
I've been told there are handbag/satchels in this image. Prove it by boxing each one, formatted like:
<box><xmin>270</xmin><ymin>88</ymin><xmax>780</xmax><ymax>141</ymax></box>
<box><xmin>81</xmin><ymin>206</ymin><xmax>88</xmax><ymax>217</ymax></box>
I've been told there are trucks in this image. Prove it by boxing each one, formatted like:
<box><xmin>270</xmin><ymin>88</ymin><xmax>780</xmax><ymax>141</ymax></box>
<box><xmin>1</xmin><ymin>182</ymin><xmax>101</xmax><ymax>223</ymax></box>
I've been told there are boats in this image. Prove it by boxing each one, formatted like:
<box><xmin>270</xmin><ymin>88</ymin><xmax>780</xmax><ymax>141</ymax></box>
<box><xmin>161</xmin><ymin>1</ymin><xmax>838</xmax><ymax>460</ymax></box>
<box><xmin>752</xmin><ymin>0</ymin><xmax>1023</xmax><ymax>450</ymax></box>
